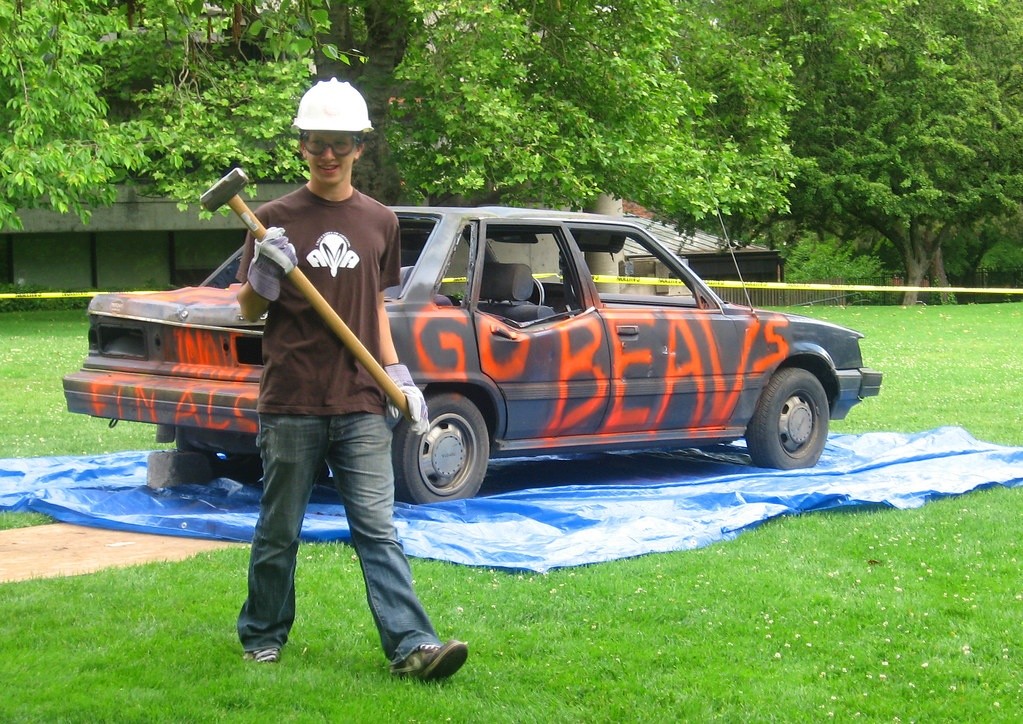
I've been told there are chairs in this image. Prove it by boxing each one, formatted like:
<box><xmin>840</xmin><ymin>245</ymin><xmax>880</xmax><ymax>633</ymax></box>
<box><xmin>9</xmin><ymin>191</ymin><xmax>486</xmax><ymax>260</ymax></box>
<box><xmin>471</xmin><ymin>262</ymin><xmax>555</xmax><ymax>324</ymax></box>
<box><xmin>382</xmin><ymin>265</ymin><xmax>452</xmax><ymax>306</ymax></box>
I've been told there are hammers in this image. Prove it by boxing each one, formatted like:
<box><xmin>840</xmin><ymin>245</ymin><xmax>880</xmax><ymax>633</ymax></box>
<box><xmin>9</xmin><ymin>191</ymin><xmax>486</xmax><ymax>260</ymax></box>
<box><xmin>200</xmin><ymin>167</ymin><xmax>417</xmax><ymax>426</ymax></box>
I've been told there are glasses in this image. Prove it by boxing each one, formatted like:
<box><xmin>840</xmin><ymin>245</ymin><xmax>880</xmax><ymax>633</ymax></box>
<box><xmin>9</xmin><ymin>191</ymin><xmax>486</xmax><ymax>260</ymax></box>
<box><xmin>299</xmin><ymin>130</ymin><xmax>358</xmax><ymax>156</ymax></box>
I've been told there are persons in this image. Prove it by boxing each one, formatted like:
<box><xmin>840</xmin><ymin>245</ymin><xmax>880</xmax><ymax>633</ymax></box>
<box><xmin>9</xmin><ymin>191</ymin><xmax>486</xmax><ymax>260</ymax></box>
<box><xmin>236</xmin><ymin>77</ymin><xmax>470</xmax><ymax>680</ymax></box>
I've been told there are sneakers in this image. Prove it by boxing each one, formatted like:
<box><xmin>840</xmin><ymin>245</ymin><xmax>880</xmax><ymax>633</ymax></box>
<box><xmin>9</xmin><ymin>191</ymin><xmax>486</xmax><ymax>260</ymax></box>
<box><xmin>243</xmin><ymin>648</ymin><xmax>280</xmax><ymax>664</ymax></box>
<box><xmin>390</xmin><ymin>639</ymin><xmax>468</xmax><ymax>682</ymax></box>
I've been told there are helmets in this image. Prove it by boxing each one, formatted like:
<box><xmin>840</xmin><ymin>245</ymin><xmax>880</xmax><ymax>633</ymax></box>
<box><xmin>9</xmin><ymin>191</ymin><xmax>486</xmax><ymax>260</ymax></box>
<box><xmin>291</xmin><ymin>76</ymin><xmax>375</xmax><ymax>133</ymax></box>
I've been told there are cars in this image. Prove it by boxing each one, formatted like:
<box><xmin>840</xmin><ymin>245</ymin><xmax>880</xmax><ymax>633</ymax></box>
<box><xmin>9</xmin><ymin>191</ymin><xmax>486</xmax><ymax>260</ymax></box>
<box><xmin>61</xmin><ymin>206</ymin><xmax>885</xmax><ymax>506</ymax></box>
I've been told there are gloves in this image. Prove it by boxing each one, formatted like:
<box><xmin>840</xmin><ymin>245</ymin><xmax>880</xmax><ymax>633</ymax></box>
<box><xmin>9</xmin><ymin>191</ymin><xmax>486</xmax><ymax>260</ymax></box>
<box><xmin>247</xmin><ymin>226</ymin><xmax>299</xmax><ymax>301</ymax></box>
<box><xmin>384</xmin><ymin>363</ymin><xmax>430</xmax><ymax>436</ymax></box>
